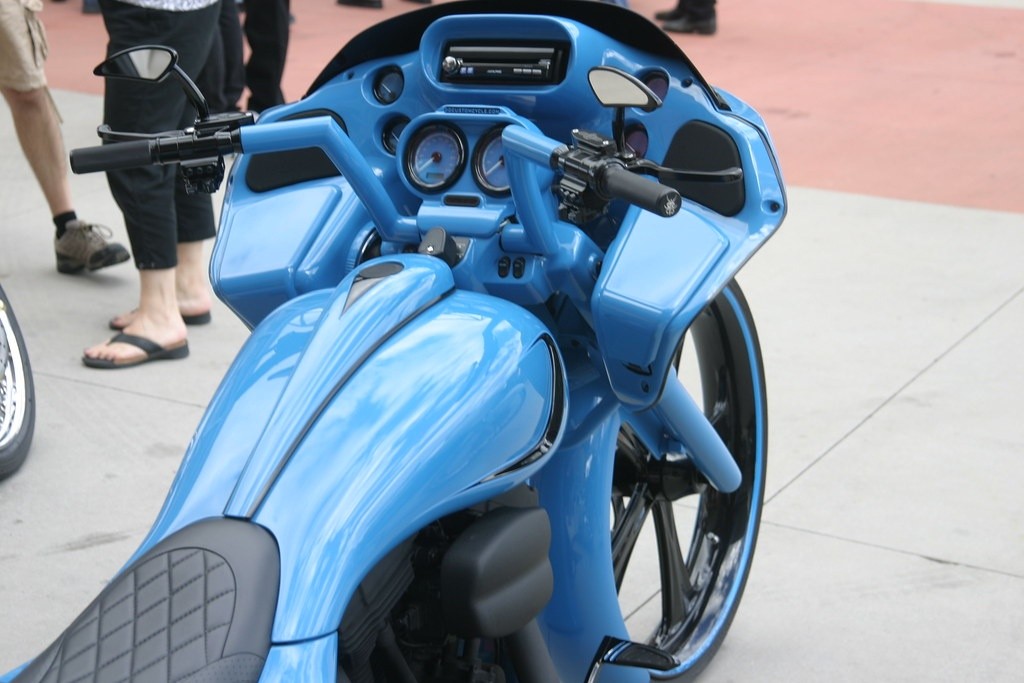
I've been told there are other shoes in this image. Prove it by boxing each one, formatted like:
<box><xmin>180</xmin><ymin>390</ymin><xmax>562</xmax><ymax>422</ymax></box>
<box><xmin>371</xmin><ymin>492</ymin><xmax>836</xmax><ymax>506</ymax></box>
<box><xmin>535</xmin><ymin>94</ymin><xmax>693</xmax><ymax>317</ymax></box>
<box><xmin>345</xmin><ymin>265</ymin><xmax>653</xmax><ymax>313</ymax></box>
<box><xmin>663</xmin><ymin>13</ymin><xmax>717</xmax><ymax>35</ymax></box>
<box><xmin>656</xmin><ymin>4</ymin><xmax>689</xmax><ymax>20</ymax></box>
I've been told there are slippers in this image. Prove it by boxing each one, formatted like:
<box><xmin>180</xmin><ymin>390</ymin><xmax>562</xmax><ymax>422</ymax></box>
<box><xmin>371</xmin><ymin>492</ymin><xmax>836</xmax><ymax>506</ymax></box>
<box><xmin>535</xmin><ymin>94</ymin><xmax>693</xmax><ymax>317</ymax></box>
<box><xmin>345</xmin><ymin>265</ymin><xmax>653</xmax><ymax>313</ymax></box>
<box><xmin>84</xmin><ymin>330</ymin><xmax>187</xmax><ymax>367</ymax></box>
<box><xmin>110</xmin><ymin>309</ymin><xmax>212</xmax><ymax>329</ymax></box>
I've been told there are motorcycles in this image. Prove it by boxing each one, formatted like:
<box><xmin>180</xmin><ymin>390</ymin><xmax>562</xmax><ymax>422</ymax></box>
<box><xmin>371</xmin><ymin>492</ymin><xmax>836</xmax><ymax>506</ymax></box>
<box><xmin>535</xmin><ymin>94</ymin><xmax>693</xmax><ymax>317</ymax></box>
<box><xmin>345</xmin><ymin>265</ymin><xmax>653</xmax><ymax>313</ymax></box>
<box><xmin>1</xmin><ymin>0</ymin><xmax>790</xmax><ymax>683</ymax></box>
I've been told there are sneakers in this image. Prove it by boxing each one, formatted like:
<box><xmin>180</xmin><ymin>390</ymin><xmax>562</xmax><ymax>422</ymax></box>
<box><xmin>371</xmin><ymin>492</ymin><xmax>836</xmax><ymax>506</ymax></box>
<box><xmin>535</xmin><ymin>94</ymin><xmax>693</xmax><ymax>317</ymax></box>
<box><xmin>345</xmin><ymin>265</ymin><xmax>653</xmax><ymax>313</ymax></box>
<box><xmin>55</xmin><ymin>220</ymin><xmax>130</xmax><ymax>273</ymax></box>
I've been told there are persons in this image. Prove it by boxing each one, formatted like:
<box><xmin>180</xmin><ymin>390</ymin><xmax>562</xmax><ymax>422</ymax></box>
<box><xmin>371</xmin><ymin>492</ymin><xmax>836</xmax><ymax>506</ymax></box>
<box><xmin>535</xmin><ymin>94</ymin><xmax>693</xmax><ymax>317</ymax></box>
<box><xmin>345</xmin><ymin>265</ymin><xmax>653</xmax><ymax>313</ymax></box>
<box><xmin>79</xmin><ymin>0</ymin><xmax>216</xmax><ymax>372</ymax></box>
<box><xmin>658</xmin><ymin>0</ymin><xmax>718</xmax><ymax>35</ymax></box>
<box><xmin>197</xmin><ymin>0</ymin><xmax>249</xmax><ymax>121</ymax></box>
<box><xmin>0</xmin><ymin>2</ymin><xmax>132</xmax><ymax>275</ymax></box>
<box><xmin>239</xmin><ymin>0</ymin><xmax>294</xmax><ymax>114</ymax></box>
